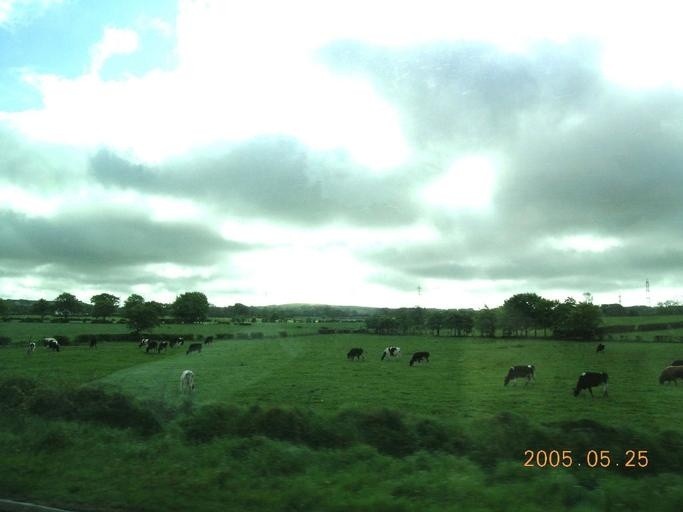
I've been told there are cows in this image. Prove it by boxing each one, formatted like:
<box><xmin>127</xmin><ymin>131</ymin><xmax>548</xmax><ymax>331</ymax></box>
<box><xmin>504</xmin><ymin>364</ymin><xmax>535</xmax><ymax>386</ymax></box>
<box><xmin>596</xmin><ymin>344</ymin><xmax>605</xmax><ymax>352</ymax></box>
<box><xmin>88</xmin><ymin>336</ymin><xmax>96</xmax><ymax>353</ymax></box>
<box><xmin>29</xmin><ymin>343</ymin><xmax>35</xmax><ymax>351</ymax></box>
<box><xmin>180</xmin><ymin>370</ymin><xmax>195</xmax><ymax>393</ymax></box>
<box><xmin>571</xmin><ymin>371</ymin><xmax>609</xmax><ymax>401</ymax></box>
<box><xmin>139</xmin><ymin>335</ymin><xmax>213</xmax><ymax>354</ymax></box>
<box><xmin>347</xmin><ymin>348</ymin><xmax>364</xmax><ymax>361</ymax></box>
<box><xmin>410</xmin><ymin>351</ymin><xmax>430</xmax><ymax>367</ymax></box>
<box><xmin>381</xmin><ymin>346</ymin><xmax>401</xmax><ymax>361</ymax></box>
<box><xmin>43</xmin><ymin>337</ymin><xmax>60</xmax><ymax>352</ymax></box>
<box><xmin>659</xmin><ymin>360</ymin><xmax>683</xmax><ymax>385</ymax></box>
